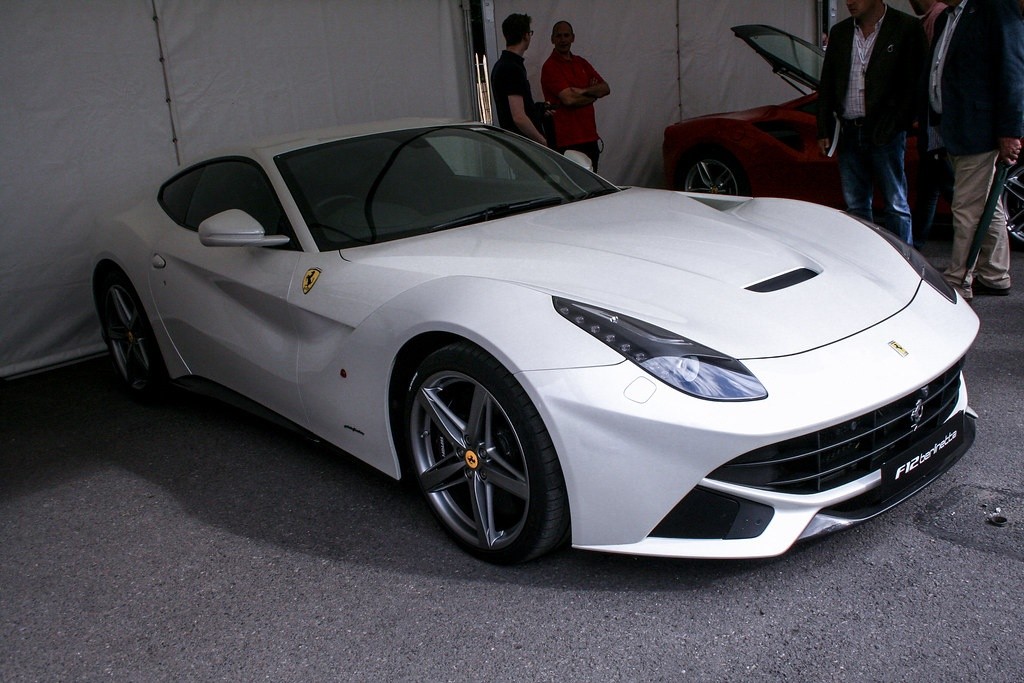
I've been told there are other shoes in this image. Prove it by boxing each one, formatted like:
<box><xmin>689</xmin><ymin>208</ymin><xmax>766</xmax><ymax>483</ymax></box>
<box><xmin>971</xmin><ymin>277</ymin><xmax>1011</xmax><ymax>295</ymax></box>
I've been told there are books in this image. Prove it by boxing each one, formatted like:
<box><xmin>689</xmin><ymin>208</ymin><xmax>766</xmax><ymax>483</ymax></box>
<box><xmin>825</xmin><ymin>113</ymin><xmax>840</xmax><ymax>157</ymax></box>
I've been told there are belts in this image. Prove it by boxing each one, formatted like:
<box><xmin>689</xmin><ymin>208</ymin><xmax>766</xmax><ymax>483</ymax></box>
<box><xmin>844</xmin><ymin>117</ymin><xmax>866</xmax><ymax>127</ymax></box>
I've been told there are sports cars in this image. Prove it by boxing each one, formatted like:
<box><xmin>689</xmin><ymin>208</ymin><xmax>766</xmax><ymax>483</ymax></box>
<box><xmin>86</xmin><ymin>115</ymin><xmax>981</xmax><ymax>571</ymax></box>
<box><xmin>660</xmin><ymin>23</ymin><xmax>1024</xmax><ymax>253</ymax></box>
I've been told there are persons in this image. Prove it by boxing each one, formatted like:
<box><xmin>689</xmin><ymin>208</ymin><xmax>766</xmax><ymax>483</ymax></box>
<box><xmin>492</xmin><ymin>14</ymin><xmax>547</xmax><ymax>182</ymax></box>
<box><xmin>908</xmin><ymin>0</ymin><xmax>1024</xmax><ymax>305</ymax></box>
<box><xmin>816</xmin><ymin>0</ymin><xmax>929</xmax><ymax>248</ymax></box>
<box><xmin>541</xmin><ymin>20</ymin><xmax>610</xmax><ymax>174</ymax></box>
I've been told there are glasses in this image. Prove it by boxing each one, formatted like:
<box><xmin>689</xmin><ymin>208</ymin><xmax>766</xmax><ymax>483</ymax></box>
<box><xmin>528</xmin><ymin>30</ymin><xmax>534</xmax><ymax>36</ymax></box>
